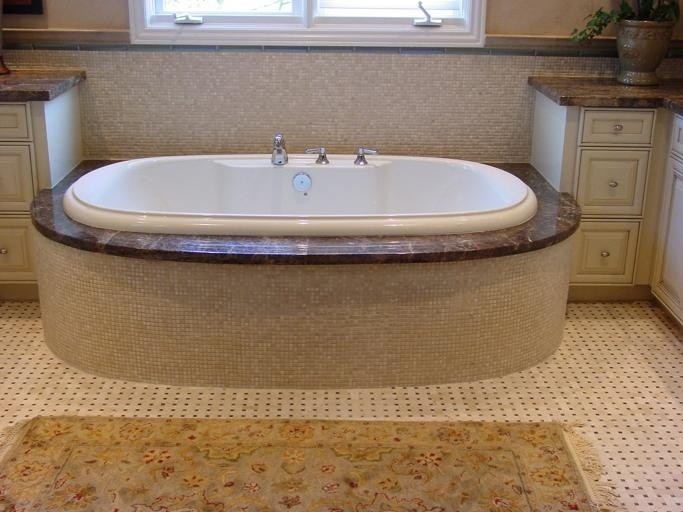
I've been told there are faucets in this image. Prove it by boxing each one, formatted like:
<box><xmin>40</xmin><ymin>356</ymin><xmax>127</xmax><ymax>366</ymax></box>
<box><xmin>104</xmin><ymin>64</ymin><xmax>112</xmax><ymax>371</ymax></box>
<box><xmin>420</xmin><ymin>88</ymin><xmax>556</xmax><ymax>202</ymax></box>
<box><xmin>272</xmin><ymin>134</ymin><xmax>288</xmax><ymax>165</ymax></box>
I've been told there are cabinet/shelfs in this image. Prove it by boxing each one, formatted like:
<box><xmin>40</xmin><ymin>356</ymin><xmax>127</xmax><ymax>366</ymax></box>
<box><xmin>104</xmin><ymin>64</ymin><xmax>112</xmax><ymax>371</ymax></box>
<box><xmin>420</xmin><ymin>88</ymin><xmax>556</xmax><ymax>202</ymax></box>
<box><xmin>636</xmin><ymin>99</ymin><xmax>683</xmax><ymax>328</ymax></box>
<box><xmin>0</xmin><ymin>83</ymin><xmax>83</xmax><ymax>300</ymax></box>
<box><xmin>529</xmin><ymin>90</ymin><xmax>672</xmax><ymax>301</ymax></box>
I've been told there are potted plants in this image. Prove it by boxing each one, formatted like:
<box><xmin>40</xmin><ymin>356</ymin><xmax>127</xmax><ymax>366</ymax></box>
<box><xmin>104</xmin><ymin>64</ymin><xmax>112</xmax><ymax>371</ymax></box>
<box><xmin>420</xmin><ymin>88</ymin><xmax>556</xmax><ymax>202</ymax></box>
<box><xmin>570</xmin><ymin>0</ymin><xmax>683</xmax><ymax>87</ymax></box>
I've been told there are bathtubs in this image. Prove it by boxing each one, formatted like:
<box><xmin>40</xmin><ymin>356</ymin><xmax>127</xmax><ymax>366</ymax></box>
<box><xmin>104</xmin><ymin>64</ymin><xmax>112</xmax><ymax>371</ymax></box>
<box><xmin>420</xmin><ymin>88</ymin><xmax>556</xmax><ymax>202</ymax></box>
<box><xmin>62</xmin><ymin>152</ymin><xmax>538</xmax><ymax>238</ymax></box>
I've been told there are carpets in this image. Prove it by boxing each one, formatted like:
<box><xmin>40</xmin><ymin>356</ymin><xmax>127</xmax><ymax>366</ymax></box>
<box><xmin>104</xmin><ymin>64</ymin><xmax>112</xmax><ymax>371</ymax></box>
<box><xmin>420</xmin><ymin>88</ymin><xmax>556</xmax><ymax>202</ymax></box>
<box><xmin>0</xmin><ymin>416</ymin><xmax>625</xmax><ymax>512</ymax></box>
